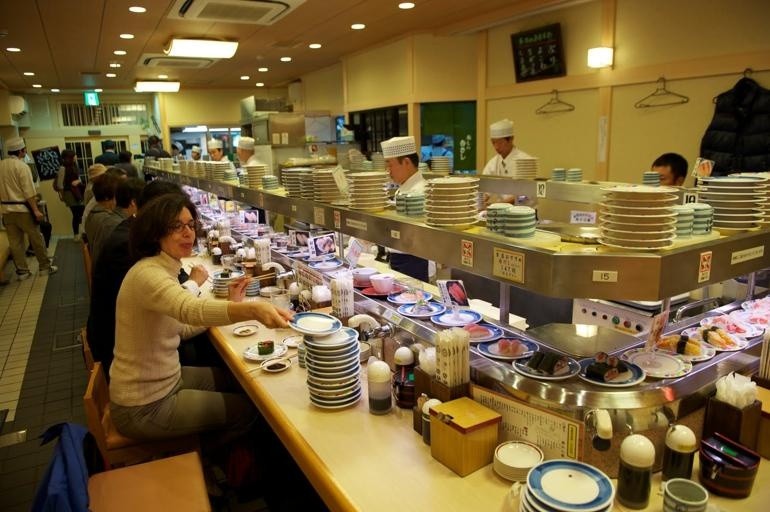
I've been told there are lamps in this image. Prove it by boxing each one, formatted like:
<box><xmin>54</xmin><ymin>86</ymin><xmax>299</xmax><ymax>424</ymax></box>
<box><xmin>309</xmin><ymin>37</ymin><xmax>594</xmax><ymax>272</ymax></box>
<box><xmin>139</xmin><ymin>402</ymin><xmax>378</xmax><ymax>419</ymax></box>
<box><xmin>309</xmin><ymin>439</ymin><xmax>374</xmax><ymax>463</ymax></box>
<box><xmin>84</xmin><ymin>450</ymin><xmax>211</xmax><ymax>511</ymax></box>
<box><xmin>84</xmin><ymin>362</ymin><xmax>206</xmax><ymax>470</ymax></box>
<box><xmin>80</xmin><ymin>327</ymin><xmax>97</xmax><ymax>368</ymax></box>
<box><xmin>80</xmin><ymin>232</ymin><xmax>93</xmax><ymax>298</ymax></box>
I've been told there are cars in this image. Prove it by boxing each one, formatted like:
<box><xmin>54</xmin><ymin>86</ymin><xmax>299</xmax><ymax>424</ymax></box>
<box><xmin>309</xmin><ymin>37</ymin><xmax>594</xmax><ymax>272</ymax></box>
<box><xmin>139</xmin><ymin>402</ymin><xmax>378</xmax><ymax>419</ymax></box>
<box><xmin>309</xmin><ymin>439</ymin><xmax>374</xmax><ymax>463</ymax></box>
<box><xmin>396</xmin><ymin>191</ymin><xmax>410</xmax><ymax>216</ymax></box>
<box><xmin>686</xmin><ymin>203</ymin><xmax>713</xmax><ymax>234</ymax></box>
<box><xmin>518</xmin><ymin>458</ymin><xmax>614</xmax><ymax>512</ymax></box>
<box><xmin>674</xmin><ymin>205</ymin><xmax>695</xmax><ymax>239</ymax></box>
<box><xmin>272</xmin><ymin>176</ymin><xmax>278</xmax><ymax>189</ymax></box>
<box><xmin>487</xmin><ymin>203</ymin><xmax>512</xmax><ymax>233</ymax></box>
<box><xmin>568</xmin><ymin>168</ymin><xmax>583</xmax><ymax>181</ymax></box>
<box><xmin>662</xmin><ymin>478</ymin><xmax>708</xmax><ymax>512</ymax></box>
<box><xmin>263</xmin><ymin>176</ymin><xmax>273</xmax><ymax>190</ymax></box>
<box><xmin>369</xmin><ymin>272</ymin><xmax>395</xmax><ymax>294</ymax></box>
<box><xmin>504</xmin><ymin>206</ymin><xmax>537</xmax><ymax>238</ymax></box>
<box><xmin>643</xmin><ymin>172</ymin><xmax>660</xmax><ymax>187</ymax></box>
<box><xmin>406</xmin><ymin>191</ymin><xmax>426</xmax><ymax>216</ymax></box>
<box><xmin>240</xmin><ymin>173</ymin><xmax>246</xmax><ymax>184</ymax></box>
<box><xmin>419</xmin><ymin>162</ymin><xmax>428</xmax><ymax>172</ymax></box>
<box><xmin>353</xmin><ymin>267</ymin><xmax>377</xmax><ymax>285</ymax></box>
<box><xmin>297</xmin><ymin>342</ymin><xmax>305</xmax><ymax>368</ymax></box>
<box><xmin>552</xmin><ymin>168</ymin><xmax>565</xmax><ymax>181</ymax></box>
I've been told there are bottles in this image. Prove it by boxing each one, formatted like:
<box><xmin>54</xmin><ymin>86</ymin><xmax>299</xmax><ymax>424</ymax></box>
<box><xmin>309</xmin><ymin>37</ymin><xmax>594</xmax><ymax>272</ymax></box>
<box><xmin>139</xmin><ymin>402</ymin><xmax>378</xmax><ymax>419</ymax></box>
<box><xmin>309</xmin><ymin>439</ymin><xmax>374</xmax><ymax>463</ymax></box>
<box><xmin>207</xmin><ymin>139</ymin><xmax>223</xmax><ymax>149</ymax></box>
<box><xmin>6</xmin><ymin>137</ymin><xmax>25</xmax><ymax>151</ymax></box>
<box><xmin>175</xmin><ymin>142</ymin><xmax>183</xmax><ymax>152</ymax></box>
<box><xmin>380</xmin><ymin>135</ymin><xmax>416</xmax><ymax>158</ymax></box>
<box><xmin>489</xmin><ymin>118</ymin><xmax>514</xmax><ymax>139</ymax></box>
<box><xmin>238</xmin><ymin>136</ymin><xmax>255</xmax><ymax>150</ymax></box>
<box><xmin>192</xmin><ymin>146</ymin><xmax>201</xmax><ymax>155</ymax></box>
<box><xmin>432</xmin><ymin>135</ymin><xmax>444</xmax><ymax>144</ymax></box>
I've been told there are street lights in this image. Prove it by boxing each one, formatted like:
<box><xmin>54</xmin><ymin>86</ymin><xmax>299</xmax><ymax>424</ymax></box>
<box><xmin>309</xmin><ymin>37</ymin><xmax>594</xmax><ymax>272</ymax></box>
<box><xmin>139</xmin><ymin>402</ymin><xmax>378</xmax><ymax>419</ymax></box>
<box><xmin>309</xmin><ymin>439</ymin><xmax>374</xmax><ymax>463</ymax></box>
<box><xmin>52</xmin><ymin>174</ymin><xmax>65</xmax><ymax>203</ymax></box>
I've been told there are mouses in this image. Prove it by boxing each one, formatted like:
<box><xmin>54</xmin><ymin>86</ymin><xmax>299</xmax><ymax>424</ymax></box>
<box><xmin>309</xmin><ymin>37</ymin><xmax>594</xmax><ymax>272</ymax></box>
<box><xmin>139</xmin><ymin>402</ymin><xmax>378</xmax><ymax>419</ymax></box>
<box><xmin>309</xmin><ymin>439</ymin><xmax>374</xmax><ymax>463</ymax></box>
<box><xmin>535</xmin><ymin>89</ymin><xmax>575</xmax><ymax>114</ymax></box>
<box><xmin>633</xmin><ymin>77</ymin><xmax>689</xmax><ymax>108</ymax></box>
<box><xmin>712</xmin><ymin>69</ymin><xmax>753</xmax><ymax>104</ymax></box>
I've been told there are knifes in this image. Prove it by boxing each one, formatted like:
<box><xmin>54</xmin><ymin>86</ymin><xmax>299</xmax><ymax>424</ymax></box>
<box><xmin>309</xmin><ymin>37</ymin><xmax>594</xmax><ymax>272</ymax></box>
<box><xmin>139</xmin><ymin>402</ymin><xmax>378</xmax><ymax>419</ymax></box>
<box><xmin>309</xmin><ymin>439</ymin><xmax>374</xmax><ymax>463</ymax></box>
<box><xmin>10</xmin><ymin>96</ymin><xmax>29</xmax><ymax>118</ymax></box>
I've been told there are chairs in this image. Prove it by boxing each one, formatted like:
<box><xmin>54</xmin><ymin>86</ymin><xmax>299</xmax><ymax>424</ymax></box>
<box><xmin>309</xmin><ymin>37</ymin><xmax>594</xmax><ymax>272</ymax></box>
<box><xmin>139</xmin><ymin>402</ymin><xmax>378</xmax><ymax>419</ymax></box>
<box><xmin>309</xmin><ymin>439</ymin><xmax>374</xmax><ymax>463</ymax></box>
<box><xmin>16</xmin><ymin>273</ymin><xmax>29</xmax><ymax>281</ymax></box>
<box><xmin>39</xmin><ymin>265</ymin><xmax>58</xmax><ymax>276</ymax></box>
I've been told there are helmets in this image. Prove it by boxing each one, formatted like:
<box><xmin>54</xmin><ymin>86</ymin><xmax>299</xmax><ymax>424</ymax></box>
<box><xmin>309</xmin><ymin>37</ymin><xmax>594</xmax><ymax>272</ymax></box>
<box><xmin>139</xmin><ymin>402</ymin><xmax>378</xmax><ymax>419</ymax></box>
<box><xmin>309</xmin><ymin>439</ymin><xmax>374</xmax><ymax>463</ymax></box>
<box><xmin>303</xmin><ymin>326</ymin><xmax>363</xmax><ymax>411</ymax></box>
<box><xmin>360</xmin><ymin>341</ymin><xmax>371</xmax><ymax>363</ymax></box>
<box><xmin>431</xmin><ymin>157</ymin><xmax>449</xmax><ymax>174</ymax></box>
<box><xmin>387</xmin><ymin>288</ymin><xmax>432</xmax><ymax>306</ymax></box>
<box><xmin>299</xmin><ymin>168</ymin><xmax>315</xmax><ymax>199</ymax></box>
<box><xmin>261</xmin><ymin>286</ymin><xmax>279</xmax><ymax>297</ymax></box>
<box><xmin>654</xmin><ymin>335</ymin><xmax>716</xmax><ymax>361</ymax></box>
<box><xmin>260</xmin><ymin>357</ymin><xmax>291</xmax><ymax>372</ymax></box>
<box><xmin>244</xmin><ymin>340</ymin><xmax>286</xmax><ymax>360</ymax></box>
<box><xmin>597</xmin><ymin>185</ymin><xmax>679</xmax><ymax>252</ymax></box>
<box><xmin>145</xmin><ymin>156</ymin><xmax>231</xmax><ymax>180</ymax></box>
<box><xmin>289</xmin><ymin>312</ymin><xmax>342</xmax><ymax>337</ymax></box>
<box><xmin>361</xmin><ymin>285</ymin><xmax>404</xmax><ymax>296</ymax></box>
<box><xmin>700</xmin><ymin>316</ymin><xmax>756</xmax><ymax>339</ymax></box>
<box><xmin>681</xmin><ymin>327</ymin><xmax>748</xmax><ymax>352</ymax></box>
<box><xmin>232</xmin><ymin>324</ymin><xmax>260</xmax><ymax>336</ymax></box>
<box><xmin>512</xmin><ymin>352</ymin><xmax>581</xmax><ymax>379</ymax></box>
<box><xmin>397</xmin><ymin>301</ymin><xmax>447</xmax><ymax>320</ymax></box>
<box><xmin>246</xmin><ymin>165</ymin><xmax>265</xmax><ymax>188</ymax></box>
<box><xmin>698</xmin><ymin>176</ymin><xmax>768</xmax><ymax>186</ymax></box>
<box><xmin>477</xmin><ymin>335</ymin><xmax>540</xmax><ymax>359</ymax></box>
<box><xmin>424</xmin><ymin>177</ymin><xmax>481</xmax><ymax>230</ymax></box>
<box><xmin>620</xmin><ymin>348</ymin><xmax>692</xmax><ymax>378</ymax></box>
<box><xmin>430</xmin><ymin>309</ymin><xmax>483</xmax><ymax>328</ymax></box>
<box><xmin>203</xmin><ymin>209</ymin><xmax>305</xmax><ymax>252</ymax></box>
<box><xmin>312</xmin><ymin>168</ymin><xmax>346</xmax><ymax>203</ymax></box>
<box><xmin>353</xmin><ymin>278</ymin><xmax>372</xmax><ymax>287</ymax></box>
<box><xmin>729</xmin><ymin>309</ymin><xmax>765</xmax><ymax>338</ymax></box>
<box><xmin>307</xmin><ymin>258</ymin><xmax>343</xmax><ymax>271</ymax></box>
<box><xmin>282</xmin><ymin>167</ymin><xmax>300</xmax><ymax>197</ymax></box>
<box><xmin>213</xmin><ymin>269</ymin><xmax>245</xmax><ymax>297</ymax></box>
<box><xmin>302</xmin><ymin>255</ymin><xmax>335</xmax><ymax>261</ymax></box>
<box><xmin>713</xmin><ymin>204</ymin><xmax>765</xmax><ymax>213</ymax></box>
<box><xmin>578</xmin><ymin>357</ymin><xmax>646</xmax><ymax>387</ymax></box>
<box><xmin>741</xmin><ymin>300</ymin><xmax>770</xmax><ymax>311</ymax></box>
<box><xmin>712</xmin><ymin>224</ymin><xmax>762</xmax><ymax>232</ymax></box>
<box><xmin>697</xmin><ymin>184</ymin><xmax>767</xmax><ymax>192</ymax></box>
<box><xmin>245</xmin><ymin>280</ymin><xmax>260</xmax><ymax>296</ymax></box>
<box><xmin>345</xmin><ymin>172</ymin><xmax>390</xmax><ymax>212</ymax></box>
<box><xmin>713</xmin><ymin>211</ymin><xmax>766</xmax><ymax>220</ymax></box>
<box><xmin>713</xmin><ymin>218</ymin><xmax>765</xmax><ymax>227</ymax></box>
<box><xmin>697</xmin><ymin>191</ymin><xmax>766</xmax><ymax>199</ymax></box>
<box><xmin>282</xmin><ymin>334</ymin><xmax>303</xmax><ymax>347</ymax></box>
<box><xmin>697</xmin><ymin>197</ymin><xmax>768</xmax><ymax>206</ymax></box>
<box><xmin>449</xmin><ymin>323</ymin><xmax>504</xmax><ymax>343</ymax></box>
<box><xmin>287</xmin><ymin>252</ymin><xmax>310</xmax><ymax>257</ymax></box>
<box><xmin>493</xmin><ymin>441</ymin><xmax>544</xmax><ymax>483</ymax></box>
<box><xmin>513</xmin><ymin>158</ymin><xmax>538</xmax><ymax>179</ymax></box>
<box><xmin>339</xmin><ymin>152</ymin><xmax>386</xmax><ymax>171</ymax></box>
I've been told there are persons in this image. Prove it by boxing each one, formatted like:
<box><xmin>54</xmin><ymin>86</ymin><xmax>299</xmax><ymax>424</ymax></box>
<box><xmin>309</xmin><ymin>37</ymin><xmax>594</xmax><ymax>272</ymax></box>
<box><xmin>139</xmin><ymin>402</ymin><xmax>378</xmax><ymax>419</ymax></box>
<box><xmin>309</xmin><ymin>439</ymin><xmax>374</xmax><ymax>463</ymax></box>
<box><xmin>53</xmin><ymin>149</ymin><xmax>84</xmax><ymax>242</ymax></box>
<box><xmin>145</xmin><ymin>135</ymin><xmax>170</xmax><ymax>158</ymax></box>
<box><xmin>113</xmin><ymin>152</ymin><xmax>137</xmax><ymax>177</ymax></box>
<box><xmin>421</xmin><ymin>134</ymin><xmax>454</xmax><ymax>167</ymax></box>
<box><xmin>172</xmin><ymin>141</ymin><xmax>183</xmax><ymax>156</ymax></box>
<box><xmin>86</xmin><ymin>180</ymin><xmax>206</xmax><ymax>365</ymax></box>
<box><xmin>21</xmin><ymin>158</ymin><xmax>52</xmax><ymax>257</ymax></box>
<box><xmin>84</xmin><ymin>172</ymin><xmax>125</xmax><ymax>252</ymax></box>
<box><xmin>95</xmin><ymin>140</ymin><xmax>120</xmax><ymax>165</ymax></box>
<box><xmin>236</xmin><ymin>137</ymin><xmax>263</xmax><ymax>172</ymax></box>
<box><xmin>90</xmin><ymin>176</ymin><xmax>148</xmax><ymax>269</ymax></box>
<box><xmin>84</xmin><ymin>163</ymin><xmax>107</xmax><ymax>207</ymax></box>
<box><xmin>206</xmin><ymin>138</ymin><xmax>235</xmax><ymax>169</ymax></box>
<box><xmin>110</xmin><ymin>192</ymin><xmax>291</xmax><ymax>492</ymax></box>
<box><xmin>81</xmin><ymin>167</ymin><xmax>128</xmax><ymax>244</ymax></box>
<box><xmin>380</xmin><ymin>135</ymin><xmax>430</xmax><ymax>205</ymax></box>
<box><xmin>644</xmin><ymin>154</ymin><xmax>690</xmax><ymax>187</ymax></box>
<box><xmin>0</xmin><ymin>137</ymin><xmax>58</xmax><ymax>281</ymax></box>
<box><xmin>482</xmin><ymin>119</ymin><xmax>535</xmax><ymax>178</ymax></box>
<box><xmin>191</xmin><ymin>145</ymin><xmax>203</xmax><ymax>161</ymax></box>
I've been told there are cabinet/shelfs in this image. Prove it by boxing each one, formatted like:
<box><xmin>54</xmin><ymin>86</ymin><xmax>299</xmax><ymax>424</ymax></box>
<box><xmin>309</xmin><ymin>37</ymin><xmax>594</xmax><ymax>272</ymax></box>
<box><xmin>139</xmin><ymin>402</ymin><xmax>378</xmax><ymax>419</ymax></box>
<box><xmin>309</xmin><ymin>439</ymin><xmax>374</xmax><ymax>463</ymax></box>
<box><xmin>396</xmin><ymin>350</ymin><xmax>415</xmax><ymax>408</ymax></box>
<box><xmin>368</xmin><ymin>362</ymin><xmax>390</xmax><ymax>414</ymax></box>
<box><xmin>300</xmin><ymin>289</ymin><xmax>311</xmax><ymax>306</ymax></box>
<box><xmin>212</xmin><ymin>248</ymin><xmax>221</xmax><ymax>264</ymax></box>
<box><xmin>661</xmin><ymin>425</ymin><xmax>697</xmax><ymax>484</ymax></box>
<box><xmin>290</xmin><ymin>282</ymin><xmax>301</xmax><ymax>309</ymax></box>
<box><xmin>618</xmin><ymin>433</ymin><xmax>656</xmax><ymax>508</ymax></box>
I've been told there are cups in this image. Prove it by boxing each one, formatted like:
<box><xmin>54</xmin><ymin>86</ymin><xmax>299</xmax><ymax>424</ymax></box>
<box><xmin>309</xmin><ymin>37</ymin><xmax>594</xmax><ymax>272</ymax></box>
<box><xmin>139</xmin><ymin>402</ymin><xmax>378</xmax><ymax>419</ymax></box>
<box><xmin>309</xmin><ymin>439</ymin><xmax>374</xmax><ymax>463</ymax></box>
<box><xmin>170</xmin><ymin>220</ymin><xmax>201</xmax><ymax>233</ymax></box>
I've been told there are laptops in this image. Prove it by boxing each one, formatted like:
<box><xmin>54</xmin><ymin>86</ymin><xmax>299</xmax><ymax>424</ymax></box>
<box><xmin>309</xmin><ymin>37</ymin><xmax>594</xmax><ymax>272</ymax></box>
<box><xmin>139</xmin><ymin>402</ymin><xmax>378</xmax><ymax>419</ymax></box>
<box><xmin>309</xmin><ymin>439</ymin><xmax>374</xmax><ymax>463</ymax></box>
<box><xmin>659</xmin><ymin>334</ymin><xmax>700</xmax><ymax>354</ymax></box>
<box><xmin>701</xmin><ymin>320</ymin><xmax>738</xmax><ymax>350</ymax></box>
<box><xmin>467</xmin><ymin>324</ymin><xmax>489</xmax><ymax>337</ymax></box>
<box><xmin>496</xmin><ymin>337</ymin><xmax>527</xmax><ymax>356</ymax></box>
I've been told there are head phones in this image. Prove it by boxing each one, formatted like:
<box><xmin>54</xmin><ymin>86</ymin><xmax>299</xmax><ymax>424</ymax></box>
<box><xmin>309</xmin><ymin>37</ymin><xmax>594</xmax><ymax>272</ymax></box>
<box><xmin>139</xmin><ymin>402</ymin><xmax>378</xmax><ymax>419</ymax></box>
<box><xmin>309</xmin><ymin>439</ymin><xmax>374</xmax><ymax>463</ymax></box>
<box><xmin>331</xmin><ymin>278</ymin><xmax>354</xmax><ymax>318</ymax></box>
<box><xmin>249</xmin><ymin>273</ymin><xmax>276</xmax><ymax>281</ymax></box>
<box><xmin>434</xmin><ymin>327</ymin><xmax>470</xmax><ymax>388</ymax></box>
<box><xmin>244</xmin><ymin>353</ymin><xmax>298</xmax><ymax>374</ymax></box>
<box><xmin>188</xmin><ymin>261</ymin><xmax>213</xmax><ymax>283</ymax></box>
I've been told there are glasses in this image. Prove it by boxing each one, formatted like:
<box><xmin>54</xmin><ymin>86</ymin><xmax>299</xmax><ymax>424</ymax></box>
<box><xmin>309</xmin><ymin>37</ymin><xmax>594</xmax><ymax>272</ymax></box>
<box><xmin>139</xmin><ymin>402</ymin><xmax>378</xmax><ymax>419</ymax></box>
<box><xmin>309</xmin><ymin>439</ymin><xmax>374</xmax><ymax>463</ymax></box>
<box><xmin>163</xmin><ymin>36</ymin><xmax>238</xmax><ymax>60</ymax></box>
<box><xmin>133</xmin><ymin>81</ymin><xmax>180</xmax><ymax>94</ymax></box>
<box><xmin>587</xmin><ymin>46</ymin><xmax>613</xmax><ymax>70</ymax></box>
